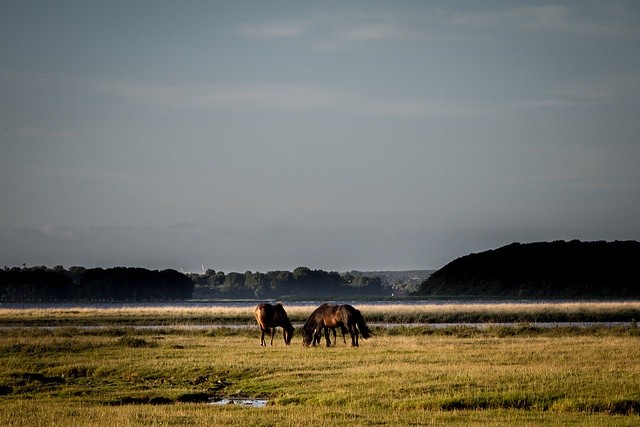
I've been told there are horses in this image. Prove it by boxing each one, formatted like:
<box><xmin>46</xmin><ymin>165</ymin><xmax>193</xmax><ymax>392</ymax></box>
<box><xmin>254</xmin><ymin>302</ymin><xmax>295</xmax><ymax>346</ymax></box>
<box><xmin>300</xmin><ymin>302</ymin><xmax>371</xmax><ymax>347</ymax></box>
